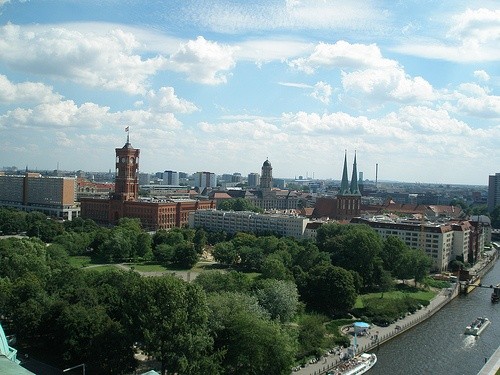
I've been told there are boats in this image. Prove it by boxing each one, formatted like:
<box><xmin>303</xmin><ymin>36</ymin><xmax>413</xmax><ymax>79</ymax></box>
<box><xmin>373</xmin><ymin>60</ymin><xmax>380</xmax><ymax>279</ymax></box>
<box><xmin>464</xmin><ymin>313</ymin><xmax>491</xmax><ymax>336</ymax></box>
<box><xmin>327</xmin><ymin>352</ymin><xmax>376</xmax><ymax>375</ymax></box>
